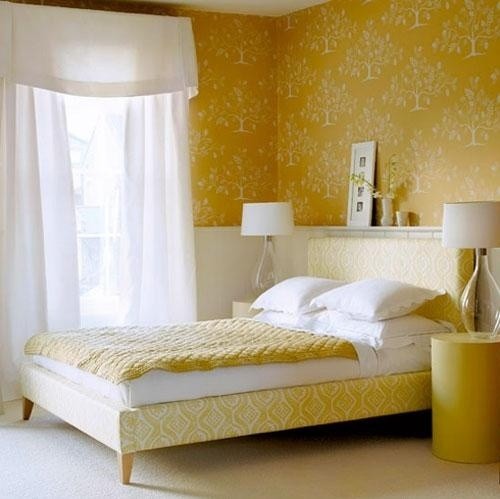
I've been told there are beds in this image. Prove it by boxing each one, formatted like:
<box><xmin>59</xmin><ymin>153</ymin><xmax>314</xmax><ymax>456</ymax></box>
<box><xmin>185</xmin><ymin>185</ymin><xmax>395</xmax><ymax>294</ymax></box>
<box><xmin>22</xmin><ymin>238</ymin><xmax>476</xmax><ymax>486</ymax></box>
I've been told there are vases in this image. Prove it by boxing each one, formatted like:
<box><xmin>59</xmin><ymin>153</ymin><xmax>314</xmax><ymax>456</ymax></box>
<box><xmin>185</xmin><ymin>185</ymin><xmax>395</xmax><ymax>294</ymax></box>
<box><xmin>380</xmin><ymin>199</ymin><xmax>394</xmax><ymax>226</ymax></box>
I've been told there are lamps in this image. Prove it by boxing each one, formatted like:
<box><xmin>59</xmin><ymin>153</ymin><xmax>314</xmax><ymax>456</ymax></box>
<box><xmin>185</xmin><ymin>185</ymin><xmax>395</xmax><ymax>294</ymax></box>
<box><xmin>240</xmin><ymin>200</ymin><xmax>294</xmax><ymax>299</ymax></box>
<box><xmin>443</xmin><ymin>199</ymin><xmax>499</xmax><ymax>340</ymax></box>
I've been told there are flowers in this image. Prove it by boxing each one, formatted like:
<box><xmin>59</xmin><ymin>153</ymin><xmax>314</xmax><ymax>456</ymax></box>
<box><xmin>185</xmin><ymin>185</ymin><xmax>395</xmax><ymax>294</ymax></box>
<box><xmin>350</xmin><ymin>154</ymin><xmax>417</xmax><ymax>199</ymax></box>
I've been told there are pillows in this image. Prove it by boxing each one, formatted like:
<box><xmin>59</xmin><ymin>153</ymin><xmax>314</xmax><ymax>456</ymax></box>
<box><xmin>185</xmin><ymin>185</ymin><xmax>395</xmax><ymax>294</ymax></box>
<box><xmin>249</xmin><ymin>275</ymin><xmax>454</xmax><ymax>353</ymax></box>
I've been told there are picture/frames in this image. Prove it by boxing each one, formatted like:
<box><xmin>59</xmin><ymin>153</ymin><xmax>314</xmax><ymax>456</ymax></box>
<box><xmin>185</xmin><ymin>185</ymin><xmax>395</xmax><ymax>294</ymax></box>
<box><xmin>346</xmin><ymin>140</ymin><xmax>377</xmax><ymax>226</ymax></box>
